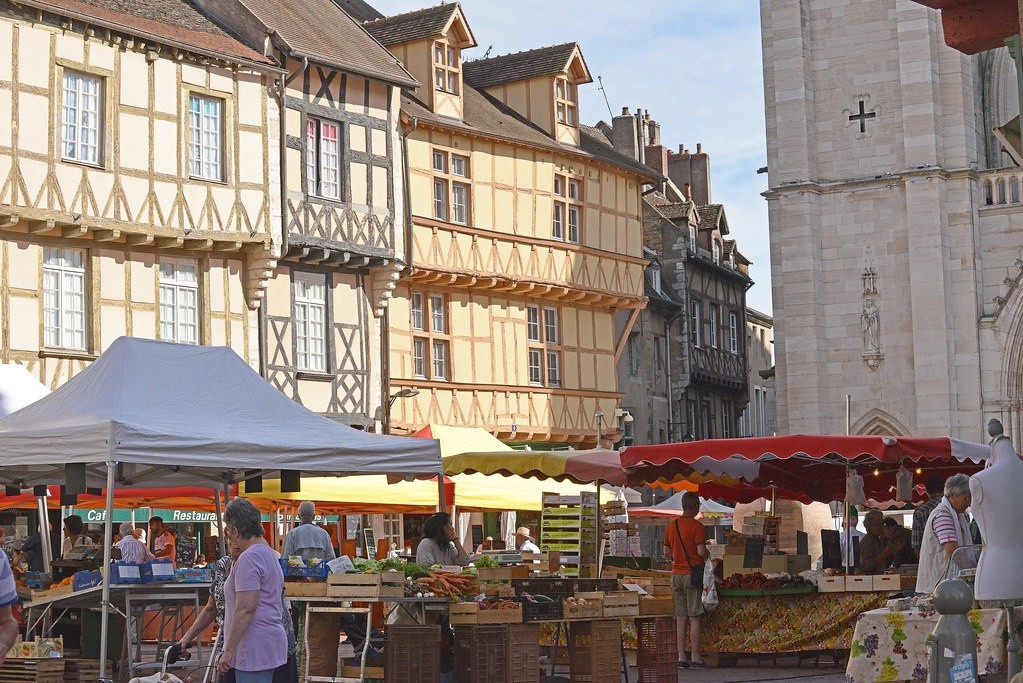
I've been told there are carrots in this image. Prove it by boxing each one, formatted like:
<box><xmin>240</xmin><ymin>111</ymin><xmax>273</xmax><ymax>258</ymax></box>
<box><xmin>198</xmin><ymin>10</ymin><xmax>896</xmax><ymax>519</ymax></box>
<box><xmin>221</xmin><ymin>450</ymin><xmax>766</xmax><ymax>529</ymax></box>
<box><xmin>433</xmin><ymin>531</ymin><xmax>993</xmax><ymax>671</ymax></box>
<box><xmin>416</xmin><ymin>568</ymin><xmax>475</xmax><ymax>597</ymax></box>
<box><xmin>485</xmin><ymin>600</ymin><xmax>519</xmax><ymax>609</ymax></box>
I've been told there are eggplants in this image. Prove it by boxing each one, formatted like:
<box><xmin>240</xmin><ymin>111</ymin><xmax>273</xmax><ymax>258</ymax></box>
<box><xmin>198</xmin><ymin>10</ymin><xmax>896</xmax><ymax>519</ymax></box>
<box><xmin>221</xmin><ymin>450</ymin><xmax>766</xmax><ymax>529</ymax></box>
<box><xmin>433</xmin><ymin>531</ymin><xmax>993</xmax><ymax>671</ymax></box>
<box><xmin>776</xmin><ymin>573</ymin><xmax>813</xmax><ymax>586</ymax></box>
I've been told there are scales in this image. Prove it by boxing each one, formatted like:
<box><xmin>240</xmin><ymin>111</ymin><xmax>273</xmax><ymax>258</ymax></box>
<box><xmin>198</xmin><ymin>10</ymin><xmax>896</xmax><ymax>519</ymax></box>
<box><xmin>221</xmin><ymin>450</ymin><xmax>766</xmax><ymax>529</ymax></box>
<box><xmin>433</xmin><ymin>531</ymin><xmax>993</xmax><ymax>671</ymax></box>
<box><xmin>464</xmin><ymin>550</ymin><xmax>524</xmax><ymax>564</ymax></box>
<box><xmin>64</xmin><ymin>544</ymin><xmax>94</xmax><ymax>561</ymax></box>
<box><xmin>83</xmin><ymin>545</ymin><xmax>123</xmax><ymax>562</ymax></box>
<box><xmin>889</xmin><ymin>564</ymin><xmax>918</xmax><ymax>576</ymax></box>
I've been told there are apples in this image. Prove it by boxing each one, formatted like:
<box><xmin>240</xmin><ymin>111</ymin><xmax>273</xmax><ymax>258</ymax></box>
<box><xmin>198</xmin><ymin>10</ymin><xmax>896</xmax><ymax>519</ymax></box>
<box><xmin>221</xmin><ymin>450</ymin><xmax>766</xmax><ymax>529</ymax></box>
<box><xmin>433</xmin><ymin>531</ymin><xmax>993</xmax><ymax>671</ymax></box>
<box><xmin>563</xmin><ymin>597</ymin><xmax>587</xmax><ymax>607</ymax></box>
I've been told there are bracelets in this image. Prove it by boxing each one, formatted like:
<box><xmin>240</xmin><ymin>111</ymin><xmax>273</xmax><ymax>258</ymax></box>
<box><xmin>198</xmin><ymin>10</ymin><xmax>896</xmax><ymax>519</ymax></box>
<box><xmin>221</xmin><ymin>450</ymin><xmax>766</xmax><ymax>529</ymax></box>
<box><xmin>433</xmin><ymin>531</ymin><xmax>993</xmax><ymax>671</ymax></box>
<box><xmin>452</xmin><ymin>536</ymin><xmax>458</xmax><ymax>541</ymax></box>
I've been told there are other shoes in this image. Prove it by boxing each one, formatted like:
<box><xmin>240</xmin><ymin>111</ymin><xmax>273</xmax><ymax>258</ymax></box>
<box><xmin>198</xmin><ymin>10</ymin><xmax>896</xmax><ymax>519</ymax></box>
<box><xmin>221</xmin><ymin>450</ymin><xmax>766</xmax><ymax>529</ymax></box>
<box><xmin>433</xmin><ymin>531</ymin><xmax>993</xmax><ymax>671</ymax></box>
<box><xmin>677</xmin><ymin>661</ymin><xmax>690</xmax><ymax>669</ymax></box>
<box><xmin>688</xmin><ymin>660</ymin><xmax>714</xmax><ymax>670</ymax></box>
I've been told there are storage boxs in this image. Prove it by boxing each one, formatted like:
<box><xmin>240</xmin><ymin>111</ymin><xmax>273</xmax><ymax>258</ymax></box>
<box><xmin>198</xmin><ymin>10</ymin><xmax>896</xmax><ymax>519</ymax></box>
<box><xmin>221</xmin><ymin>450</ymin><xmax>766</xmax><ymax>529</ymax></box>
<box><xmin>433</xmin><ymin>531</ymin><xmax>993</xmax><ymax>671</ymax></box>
<box><xmin>453</xmin><ymin>624</ymin><xmax>540</xmax><ymax>683</ymax></box>
<box><xmin>326</xmin><ymin>572</ymin><xmax>406</xmax><ymax>598</ymax></box>
<box><xmin>478</xmin><ymin>564</ymin><xmax>529</xmax><ymax>580</ymax></box>
<box><xmin>140</xmin><ymin>559</ymin><xmax>175</xmax><ymax>585</ymax></box>
<box><xmin>565</xmin><ymin>591</ymin><xmax>640</xmax><ymax>619</ymax></box>
<box><xmin>74</xmin><ymin>559</ymin><xmax>142</xmax><ymax>593</ymax></box>
<box><xmin>569</xmin><ymin>619</ymin><xmax>621</xmax><ymax>683</ymax></box>
<box><xmin>6</xmin><ymin>634</ymin><xmax>63</xmax><ymax>659</ymax></box>
<box><xmin>512</xmin><ymin>575</ymin><xmax>618</xmax><ymax>598</ymax></box>
<box><xmin>873</xmin><ymin>574</ymin><xmax>901</xmax><ymax>591</ymax></box>
<box><xmin>901</xmin><ymin>576</ymin><xmax>917</xmax><ymax>589</ymax></box>
<box><xmin>723</xmin><ymin>511</ymin><xmax>812</xmax><ymax>582</ymax></box>
<box><xmin>845</xmin><ymin>574</ymin><xmax>872</xmax><ymax>592</ymax></box>
<box><xmin>600</xmin><ymin>501</ymin><xmax>627</xmax><ymax>557</ymax></box>
<box><xmin>283</xmin><ymin>580</ymin><xmax>327</xmax><ymax>596</ymax></box>
<box><xmin>499</xmin><ymin>596</ymin><xmax>564</xmax><ymax>621</ymax></box>
<box><xmin>634</xmin><ymin>618</ymin><xmax>679</xmax><ymax>683</ymax></box>
<box><xmin>30</xmin><ymin>582</ymin><xmax>73</xmax><ymax>604</ymax></box>
<box><xmin>174</xmin><ymin>568</ymin><xmax>213</xmax><ymax>584</ymax></box>
<box><xmin>706</xmin><ymin>544</ymin><xmax>724</xmax><ymax>561</ymax></box>
<box><xmin>383</xmin><ymin>623</ymin><xmax>442</xmax><ymax>683</ymax></box>
<box><xmin>448</xmin><ymin>601</ymin><xmax>523</xmax><ymax>624</ymax></box>
<box><xmin>277</xmin><ymin>557</ymin><xmax>327</xmax><ymax>580</ymax></box>
<box><xmin>619</xmin><ymin>569</ymin><xmax>676</xmax><ymax>616</ymax></box>
<box><xmin>818</xmin><ymin>572</ymin><xmax>845</xmax><ymax>592</ymax></box>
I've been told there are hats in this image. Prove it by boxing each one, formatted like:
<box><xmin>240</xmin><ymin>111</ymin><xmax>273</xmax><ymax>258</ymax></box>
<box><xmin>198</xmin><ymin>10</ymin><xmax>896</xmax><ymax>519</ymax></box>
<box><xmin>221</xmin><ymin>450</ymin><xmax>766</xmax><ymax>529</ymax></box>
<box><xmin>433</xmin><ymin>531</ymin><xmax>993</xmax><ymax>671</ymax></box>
<box><xmin>512</xmin><ymin>526</ymin><xmax>534</xmax><ymax>542</ymax></box>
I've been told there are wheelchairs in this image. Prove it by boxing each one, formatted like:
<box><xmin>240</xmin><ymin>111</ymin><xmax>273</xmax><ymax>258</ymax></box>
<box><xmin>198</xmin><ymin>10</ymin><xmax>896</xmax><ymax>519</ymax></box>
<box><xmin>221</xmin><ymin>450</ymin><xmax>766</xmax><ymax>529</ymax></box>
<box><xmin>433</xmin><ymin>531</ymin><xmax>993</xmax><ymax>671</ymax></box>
<box><xmin>99</xmin><ymin>643</ymin><xmax>224</xmax><ymax>683</ymax></box>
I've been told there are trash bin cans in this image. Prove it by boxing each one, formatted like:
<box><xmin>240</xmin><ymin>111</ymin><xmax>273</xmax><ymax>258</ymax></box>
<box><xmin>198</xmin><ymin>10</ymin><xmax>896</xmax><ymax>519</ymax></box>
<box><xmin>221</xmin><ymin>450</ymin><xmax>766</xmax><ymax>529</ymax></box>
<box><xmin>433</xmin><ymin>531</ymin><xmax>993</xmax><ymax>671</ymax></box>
<box><xmin>80</xmin><ymin>606</ymin><xmax>125</xmax><ymax>660</ymax></box>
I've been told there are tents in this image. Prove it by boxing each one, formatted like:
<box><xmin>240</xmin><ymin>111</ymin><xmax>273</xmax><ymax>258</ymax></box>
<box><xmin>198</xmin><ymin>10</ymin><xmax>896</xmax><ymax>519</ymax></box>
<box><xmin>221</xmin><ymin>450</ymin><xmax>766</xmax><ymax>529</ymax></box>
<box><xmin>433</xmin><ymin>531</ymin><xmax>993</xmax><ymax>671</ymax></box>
<box><xmin>619</xmin><ymin>434</ymin><xmax>1022</xmax><ymax>576</ymax></box>
<box><xmin>240</xmin><ymin>425</ymin><xmax>735</xmax><ymax>577</ymax></box>
<box><xmin>0</xmin><ymin>484</ymin><xmax>238</xmax><ymax>554</ymax></box>
<box><xmin>0</xmin><ymin>336</ymin><xmax>446</xmax><ymax>683</ymax></box>
<box><xmin>0</xmin><ymin>363</ymin><xmax>51</xmax><ymax>418</ymax></box>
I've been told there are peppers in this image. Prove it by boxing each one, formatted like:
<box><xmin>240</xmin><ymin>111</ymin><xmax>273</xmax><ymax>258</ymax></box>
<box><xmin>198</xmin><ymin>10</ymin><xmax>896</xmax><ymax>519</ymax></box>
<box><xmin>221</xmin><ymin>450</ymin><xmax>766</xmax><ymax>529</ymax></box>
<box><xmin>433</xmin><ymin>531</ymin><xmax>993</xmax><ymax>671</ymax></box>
<box><xmin>719</xmin><ymin>571</ymin><xmax>780</xmax><ymax>589</ymax></box>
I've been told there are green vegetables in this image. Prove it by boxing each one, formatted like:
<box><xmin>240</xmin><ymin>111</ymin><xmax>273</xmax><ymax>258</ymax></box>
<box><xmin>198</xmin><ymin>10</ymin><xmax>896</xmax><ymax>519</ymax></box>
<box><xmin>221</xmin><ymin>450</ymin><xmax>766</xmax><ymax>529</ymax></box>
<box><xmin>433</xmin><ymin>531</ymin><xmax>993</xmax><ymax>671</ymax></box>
<box><xmin>462</xmin><ymin>555</ymin><xmax>504</xmax><ymax>598</ymax></box>
<box><xmin>351</xmin><ymin>556</ymin><xmax>407</xmax><ymax>573</ymax></box>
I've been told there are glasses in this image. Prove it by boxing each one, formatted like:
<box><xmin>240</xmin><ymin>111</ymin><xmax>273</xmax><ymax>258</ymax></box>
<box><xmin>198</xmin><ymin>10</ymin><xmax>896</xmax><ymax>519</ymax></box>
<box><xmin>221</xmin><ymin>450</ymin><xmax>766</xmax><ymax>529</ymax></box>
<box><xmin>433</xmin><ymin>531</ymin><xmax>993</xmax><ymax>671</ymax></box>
<box><xmin>64</xmin><ymin>524</ymin><xmax>68</xmax><ymax>529</ymax></box>
<box><xmin>872</xmin><ymin>521</ymin><xmax>887</xmax><ymax>529</ymax></box>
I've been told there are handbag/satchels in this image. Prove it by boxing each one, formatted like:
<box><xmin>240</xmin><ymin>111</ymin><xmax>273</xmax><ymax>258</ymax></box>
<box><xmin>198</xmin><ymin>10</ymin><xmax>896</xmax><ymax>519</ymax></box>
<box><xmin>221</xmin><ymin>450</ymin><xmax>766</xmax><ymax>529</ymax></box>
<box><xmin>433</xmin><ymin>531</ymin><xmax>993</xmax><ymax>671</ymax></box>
<box><xmin>702</xmin><ymin>558</ymin><xmax>719</xmax><ymax>611</ymax></box>
<box><xmin>690</xmin><ymin>565</ymin><xmax>704</xmax><ymax>587</ymax></box>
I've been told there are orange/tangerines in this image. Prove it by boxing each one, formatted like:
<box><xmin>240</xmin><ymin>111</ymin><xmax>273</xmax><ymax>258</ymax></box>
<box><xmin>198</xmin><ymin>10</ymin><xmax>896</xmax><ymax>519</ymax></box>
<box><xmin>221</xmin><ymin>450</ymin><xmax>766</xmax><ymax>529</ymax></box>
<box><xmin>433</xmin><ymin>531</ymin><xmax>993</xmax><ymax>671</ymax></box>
<box><xmin>49</xmin><ymin>575</ymin><xmax>75</xmax><ymax>589</ymax></box>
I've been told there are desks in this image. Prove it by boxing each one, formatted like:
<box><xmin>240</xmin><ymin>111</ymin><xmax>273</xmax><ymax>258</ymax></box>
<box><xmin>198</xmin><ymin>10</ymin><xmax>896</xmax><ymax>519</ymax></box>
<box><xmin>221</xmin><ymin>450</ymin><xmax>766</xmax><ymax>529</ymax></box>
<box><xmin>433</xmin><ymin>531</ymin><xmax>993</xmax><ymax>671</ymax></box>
<box><xmin>844</xmin><ymin>604</ymin><xmax>1007</xmax><ymax>683</ymax></box>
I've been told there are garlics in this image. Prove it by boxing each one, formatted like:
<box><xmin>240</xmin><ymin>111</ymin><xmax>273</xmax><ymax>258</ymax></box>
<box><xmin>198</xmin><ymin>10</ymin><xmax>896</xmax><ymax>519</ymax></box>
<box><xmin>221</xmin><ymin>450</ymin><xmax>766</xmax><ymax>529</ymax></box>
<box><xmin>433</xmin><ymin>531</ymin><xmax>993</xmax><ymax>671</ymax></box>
<box><xmin>388</xmin><ymin>568</ymin><xmax>435</xmax><ymax>597</ymax></box>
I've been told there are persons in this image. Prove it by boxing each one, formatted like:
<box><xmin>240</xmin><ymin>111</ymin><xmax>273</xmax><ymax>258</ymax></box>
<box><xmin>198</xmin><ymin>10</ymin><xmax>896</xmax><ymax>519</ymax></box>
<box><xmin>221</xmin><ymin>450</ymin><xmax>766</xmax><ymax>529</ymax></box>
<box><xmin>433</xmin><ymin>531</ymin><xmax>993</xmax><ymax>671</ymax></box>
<box><xmin>0</xmin><ymin>516</ymin><xmax>230</xmax><ymax>573</ymax></box>
<box><xmin>177</xmin><ymin>526</ymin><xmax>299</xmax><ymax>683</ymax></box>
<box><xmin>340</xmin><ymin>601</ymin><xmax>385</xmax><ymax>653</ymax></box>
<box><xmin>415</xmin><ymin>512</ymin><xmax>471</xmax><ymax>683</ymax></box>
<box><xmin>217</xmin><ymin>499</ymin><xmax>287</xmax><ymax>683</ymax></box>
<box><xmin>280</xmin><ymin>501</ymin><xmax>335</xmax><ymax>560</ymax></box>
<box><xmin>837</xmin><ymin>473</ymin><xmax>982</xmax><ymax>594</ymax></box>
<box><xmin>512</xmin><ymin>527</ymin><xmax>540</xmax><ymax>573</ymax></box>
<box><xmin>969</xmin><ymin>438</ymin><xmax>1023</xmax><ymax>598</ymax></box>
<box><xmin>0</xmin><ymin>547</ymin><xmax>17</xmax><ymax>665</ymax></box>
<box><xmin>664</xmin><ymin>492</ymin><xmax>709</xmax><ymax>669</ymax></box>
<box><xmin>476</xmin><ymin>536</ymin><xmax>493</xmax><ymax>554</ymax></box>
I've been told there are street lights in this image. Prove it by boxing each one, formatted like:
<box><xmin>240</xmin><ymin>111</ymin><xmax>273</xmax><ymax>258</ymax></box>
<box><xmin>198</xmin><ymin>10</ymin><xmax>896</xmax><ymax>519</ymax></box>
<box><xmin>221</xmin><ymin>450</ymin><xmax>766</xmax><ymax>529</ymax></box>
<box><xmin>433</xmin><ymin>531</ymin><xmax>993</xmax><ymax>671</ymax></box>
<box><xmin>668</xmin><ymin>424</ymin><xmax>694</xmax><ymax>443</ymax></box>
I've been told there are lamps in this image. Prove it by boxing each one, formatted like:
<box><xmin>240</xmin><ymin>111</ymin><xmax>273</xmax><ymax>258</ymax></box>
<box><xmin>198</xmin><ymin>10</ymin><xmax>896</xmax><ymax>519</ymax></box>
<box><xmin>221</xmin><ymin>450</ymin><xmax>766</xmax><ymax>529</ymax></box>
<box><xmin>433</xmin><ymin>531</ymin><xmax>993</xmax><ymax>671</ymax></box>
<box><xmin>386</xmin><ymin>388</ymin><xmax>420</xmax><ymax>420</ymax></box>
<box><xmin>895</xmin><ymin>463</ymin><xmax>913</xmax><ymax>503</ymax></box>
<box><xmin>616</xmin><ymin>409</ymin><xmax>634</xmax><ymax>427</ymax></box>
<box><xmin>844</xmin><ymin>470</ymin><xmax>866</xmax><ymax>505</ymax></box>
